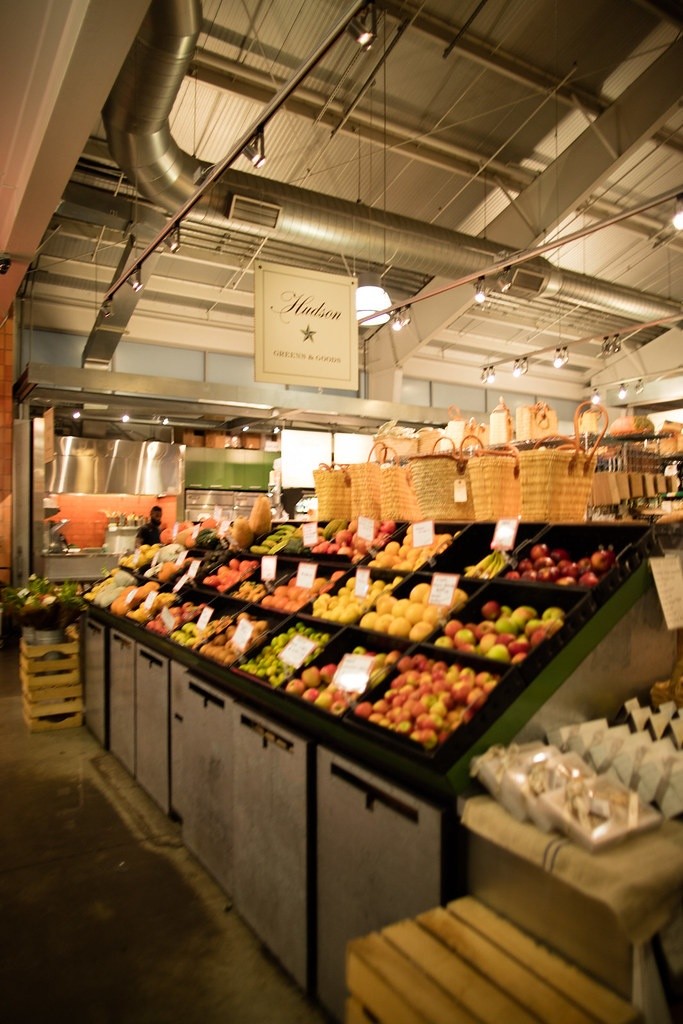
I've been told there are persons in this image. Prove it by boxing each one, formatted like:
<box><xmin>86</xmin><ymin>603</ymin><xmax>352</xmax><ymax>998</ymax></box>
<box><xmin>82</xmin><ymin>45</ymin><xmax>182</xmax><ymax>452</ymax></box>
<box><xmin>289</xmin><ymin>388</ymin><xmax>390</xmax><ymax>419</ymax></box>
<box><xmin>135</xmin><ymin>506</ymin><xmax>167</xmax><ymax>550</ymax></box>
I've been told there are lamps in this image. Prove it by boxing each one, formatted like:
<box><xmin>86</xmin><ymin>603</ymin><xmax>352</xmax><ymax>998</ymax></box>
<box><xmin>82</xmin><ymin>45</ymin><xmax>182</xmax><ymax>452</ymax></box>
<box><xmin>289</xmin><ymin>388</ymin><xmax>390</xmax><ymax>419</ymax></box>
<box><xmin>473</xmin><ymin>275</ymin><xmax>486</xmax><ymax>303</ymax></box>
<box><xmin>672</xmin><ymin>195</ymin><xmax>683</xmax><ymax>230</ymax></box>
<box><xmin>100</xmin><ymin>299</ymin><xmax>114</xmax><ymax>318</ymax></box>
<box><xmin>345</xmin><ymin>23</ymin><xmax>391</xmax><ymax>329</ymax></box>
<box><xmin>480</xmin><ymin>332</ymin><xmax>646</xmax><ymax>404</ymax></box>
<box><xmin>241</xmin><ymin>129</ymin><xmax>266</xmax><ymax>168</ymax></box>
<box><xmin>162</xmin><ymin>222</ymin><xmax>181</xmax><ymax>254</ymax></box>
<box><xmin>126</xmin><ymin>267</ymin><xmax>144</xmax><ymax>293</ymax></box>
<box><xmin>390</xmin><ymin>303</ymin><xmax>402</xmax><ymax>331</ymax></box>
<box><xmin>400</xmin><ymin>304</ymin><xmax>411</xmax><ymax>327</ymax></box>
<box><xmin>497</xmin><ymin>265</ymin><xmax>513</xmax><ymax>294</ymax></box>
<box><xmin>346</xmin><ymin>5</ymin><xmax>377</xmax><ymax>51</ymax></box>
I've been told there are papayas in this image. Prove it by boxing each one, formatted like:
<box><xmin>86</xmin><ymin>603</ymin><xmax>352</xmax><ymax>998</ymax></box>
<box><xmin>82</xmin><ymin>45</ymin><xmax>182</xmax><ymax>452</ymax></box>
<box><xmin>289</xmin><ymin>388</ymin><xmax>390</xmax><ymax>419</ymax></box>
<box><xmin>323</xmin><ymin>519</ymin><xmax>348</xmax><ymax>539</ymax></box>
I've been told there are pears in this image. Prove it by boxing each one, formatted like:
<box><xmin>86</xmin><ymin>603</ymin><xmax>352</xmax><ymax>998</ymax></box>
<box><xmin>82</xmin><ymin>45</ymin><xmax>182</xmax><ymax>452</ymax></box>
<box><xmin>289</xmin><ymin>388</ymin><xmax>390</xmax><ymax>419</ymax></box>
<box><xmin>313</xmin><ymin>524</ymin><xmax>472</xmax><ymax>643</ymax></box>
<box><xmin>311</xmin><ymin>518</ymin><xmax>396</xmax><ymax>562</ymax></box>
<box><xmin>171</xmin><ymin>622</ymin><xmax>203</xmax><ymax>647</ymax></box>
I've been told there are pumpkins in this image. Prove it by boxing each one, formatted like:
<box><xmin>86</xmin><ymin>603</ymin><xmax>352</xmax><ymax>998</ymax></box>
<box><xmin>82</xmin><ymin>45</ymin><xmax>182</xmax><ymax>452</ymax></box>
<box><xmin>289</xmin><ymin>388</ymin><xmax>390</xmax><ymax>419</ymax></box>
<box><xmin>160</xmin><ymin>496</ymin><xmax>272</xmax><ymax>549</ymax></box>
<box><xmin>84</xmin><ymin>542</ymin><xmax>194</xmax><ymax>622</ymax></box>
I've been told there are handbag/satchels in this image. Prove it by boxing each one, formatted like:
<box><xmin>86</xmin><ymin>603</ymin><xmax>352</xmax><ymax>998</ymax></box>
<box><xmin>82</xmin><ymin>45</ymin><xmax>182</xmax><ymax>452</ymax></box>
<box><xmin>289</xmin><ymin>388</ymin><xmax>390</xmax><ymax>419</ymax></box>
<box><xmin>381</xmin><ymin>446</ymin><xmax>407</xmax><ymax>520</ymax></box>
<box><xmin>465</xmin><ymin>438</ymin><xmax>520</xmax><ymax>523</ymax></box>
<box><xmin>349</xmin><ymin>438</ymin><xmax>390</xmax><ymax>521</ymax></box>
<box><xmin>313</xmin><ymin>463</ymin><xmax>349</xmax><ymax>521</ymax></box>
<box><xmin>514</xmin><ymin>400</ymin><xmax>609</xmax><ymax>522</ymax></box>
<box><xmin>404</xmin><ymin>436</ymin><xmax>477</xmax><ymax>522</ymax></box>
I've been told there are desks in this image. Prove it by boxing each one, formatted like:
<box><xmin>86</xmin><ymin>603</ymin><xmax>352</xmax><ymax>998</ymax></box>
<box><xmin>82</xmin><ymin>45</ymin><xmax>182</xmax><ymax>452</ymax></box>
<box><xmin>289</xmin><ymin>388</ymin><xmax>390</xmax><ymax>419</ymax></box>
<box><xmin>47</xmin><ymin>547</ymin><xmax>120</xmax><ymax>583</ymax></box>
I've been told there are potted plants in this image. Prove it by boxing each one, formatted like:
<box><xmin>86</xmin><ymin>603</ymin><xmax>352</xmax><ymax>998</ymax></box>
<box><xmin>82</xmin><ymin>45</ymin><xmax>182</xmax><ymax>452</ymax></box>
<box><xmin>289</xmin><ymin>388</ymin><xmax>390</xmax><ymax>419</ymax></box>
<box><xmin>0</xmin><ymin>573</ymin><xmax>85</xmax><ymax>675</ymax></box>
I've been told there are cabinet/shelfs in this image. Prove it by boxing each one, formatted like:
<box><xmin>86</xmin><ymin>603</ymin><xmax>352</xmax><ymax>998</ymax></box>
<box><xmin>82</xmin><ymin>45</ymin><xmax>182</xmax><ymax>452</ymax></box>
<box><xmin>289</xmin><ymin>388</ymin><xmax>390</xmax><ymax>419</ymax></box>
<box><xmin>80</xmin><ymin>616</ymin><xmax>447</xmax><ymax>1022</ymax></box>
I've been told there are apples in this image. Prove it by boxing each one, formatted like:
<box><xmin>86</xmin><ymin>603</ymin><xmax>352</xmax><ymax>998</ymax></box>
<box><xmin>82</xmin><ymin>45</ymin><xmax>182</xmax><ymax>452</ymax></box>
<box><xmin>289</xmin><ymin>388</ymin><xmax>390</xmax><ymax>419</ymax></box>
<box><xmin>260</xmin><ymin>570</ymin><xmax>343</xmax><ymax>613</ymax></box>
<box><xmin>202</xmin><ymin>559</ymin><xmax>258</xmax><ymax>593</ymax></box>
<box><xmin>432</xmin><ymin>543</ymin><xmax>616</xmax><ymax>666</ymax></box>
<box><xmin>283</xmin><ymin>646</ymin><xmax>500</xmax><ymax>751</ymax></box>
<box><xmin>145</xmin><ymin>600</ymin><xmax>206</xmax><ymax>636</ymax></box>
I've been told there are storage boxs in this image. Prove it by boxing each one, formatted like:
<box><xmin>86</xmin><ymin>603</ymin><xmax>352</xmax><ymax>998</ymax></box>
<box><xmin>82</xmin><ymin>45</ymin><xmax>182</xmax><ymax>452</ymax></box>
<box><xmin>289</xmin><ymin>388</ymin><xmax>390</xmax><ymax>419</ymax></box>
<box><xmin>83</xmin><ymin>517</ymin><xmax>669</xmax><ymax>806</ymax></box>
<box><xmin>469</xmin><ymin>830</ymin><xmax>634</xmax><ymax>999</ymax></box>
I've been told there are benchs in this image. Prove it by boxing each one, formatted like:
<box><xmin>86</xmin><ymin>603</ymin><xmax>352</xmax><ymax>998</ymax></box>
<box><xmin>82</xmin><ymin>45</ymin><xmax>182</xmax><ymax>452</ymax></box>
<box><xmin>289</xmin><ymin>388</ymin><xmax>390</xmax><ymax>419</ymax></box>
<box><xmin>345</xmin><ymin>895</ymin><xmax>644</xmax><ymax>1024</ymax></box>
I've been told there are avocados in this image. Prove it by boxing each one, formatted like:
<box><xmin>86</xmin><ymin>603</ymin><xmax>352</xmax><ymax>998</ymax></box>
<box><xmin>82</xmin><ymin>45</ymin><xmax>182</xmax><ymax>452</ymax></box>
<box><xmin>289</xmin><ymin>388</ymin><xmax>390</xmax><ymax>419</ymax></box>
<box><xmin>169</xmin><ymin>549</ymin><xmax>235</xmax><ymax>585</ymax></box>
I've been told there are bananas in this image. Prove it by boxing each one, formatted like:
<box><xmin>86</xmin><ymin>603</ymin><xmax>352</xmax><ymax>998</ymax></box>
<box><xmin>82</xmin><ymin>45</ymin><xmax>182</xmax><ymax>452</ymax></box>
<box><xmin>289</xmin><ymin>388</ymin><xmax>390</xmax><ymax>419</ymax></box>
<box><xmin>463</xmin><ymin>549</ymin><xmax>510</xmax><ymax>581</ymax></box>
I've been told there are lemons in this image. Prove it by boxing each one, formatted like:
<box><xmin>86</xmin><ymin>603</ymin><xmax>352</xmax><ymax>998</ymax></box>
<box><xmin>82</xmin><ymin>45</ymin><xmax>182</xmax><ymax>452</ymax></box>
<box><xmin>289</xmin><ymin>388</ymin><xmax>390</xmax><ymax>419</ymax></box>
<box><xmin>238</xmin><ymin>623</ymin><xmax>334</xmax><ymax>687</ymax></box>
